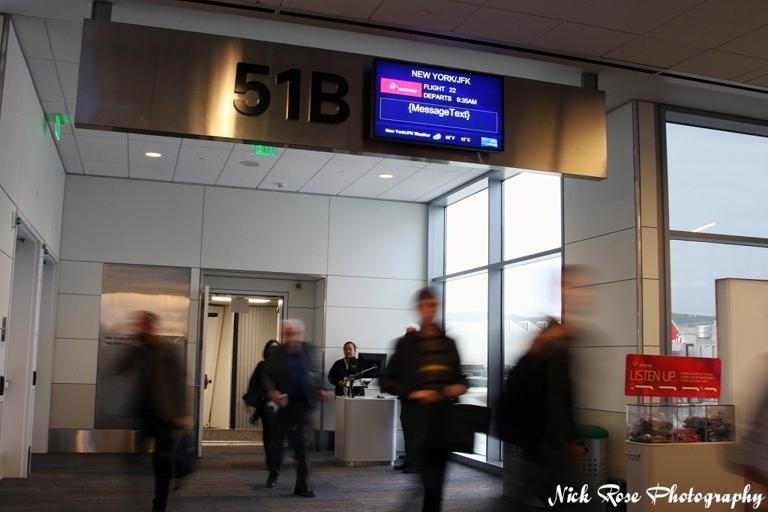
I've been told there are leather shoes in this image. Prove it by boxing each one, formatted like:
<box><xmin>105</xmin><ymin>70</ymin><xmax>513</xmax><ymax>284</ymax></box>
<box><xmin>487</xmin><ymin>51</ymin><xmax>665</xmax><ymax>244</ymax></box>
<box><xmin>295</xmin><ymin>485</ymin><xmax>316</xmax><ymax>497</ymax></box>
<box><xmin>268</xmin><ymin>472</ymin><xmax>277</xmax><ymax>488</ymax></box>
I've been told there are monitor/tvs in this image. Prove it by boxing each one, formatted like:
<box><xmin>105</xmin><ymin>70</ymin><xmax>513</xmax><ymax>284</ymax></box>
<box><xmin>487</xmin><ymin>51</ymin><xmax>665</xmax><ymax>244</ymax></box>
<box><xmin>368</xmin><ymin>57</ymin><xmax>505</xmax><ymax>152</ymax></box>
<box><xmin>358</xmin><ymin>353</ymin><xmax>387</xmax><ymax>377</ymax></box>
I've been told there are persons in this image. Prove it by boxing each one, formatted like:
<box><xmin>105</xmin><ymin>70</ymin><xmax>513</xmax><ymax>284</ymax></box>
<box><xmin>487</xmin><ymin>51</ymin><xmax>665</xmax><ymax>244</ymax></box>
<box><xmin>245</xmin><ymin>339</ymin><xmax>282</xmax><ymax>472</ymax></box>
<box><xmin>390</xmin><ymin>325</ymin><xmax>420</xmax><ymax>474</ymax></box>
<box><xmin>327</xmin><ymin>339</ymin><xmax>372</xmax><ymax>396</ymax></box>
<box><xmin>110</xmin><ymin>309</ymin><xmax>194</xmax><ymax>512</ymax></box>
<box><xmin>377</xmin><ymin>287</ymin><xmax>470</xmax><ymax>512</ymax></box>
<box><xmin>260</xmin><ymin>318</ymin><xmax>335</xmax><ymax>499</ymax></box>
<box><xmin>494</xmin><ymin>265</ymin><xmax>616</xmax><ymax>511</ymax></box>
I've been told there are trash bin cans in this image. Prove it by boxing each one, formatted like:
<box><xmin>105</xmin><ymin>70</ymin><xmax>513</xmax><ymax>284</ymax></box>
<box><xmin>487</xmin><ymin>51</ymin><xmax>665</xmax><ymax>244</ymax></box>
<box><xmin>572</xmin><ymin>424</ymin><xmax>610</xmax><ymax>490</ymax></box>
